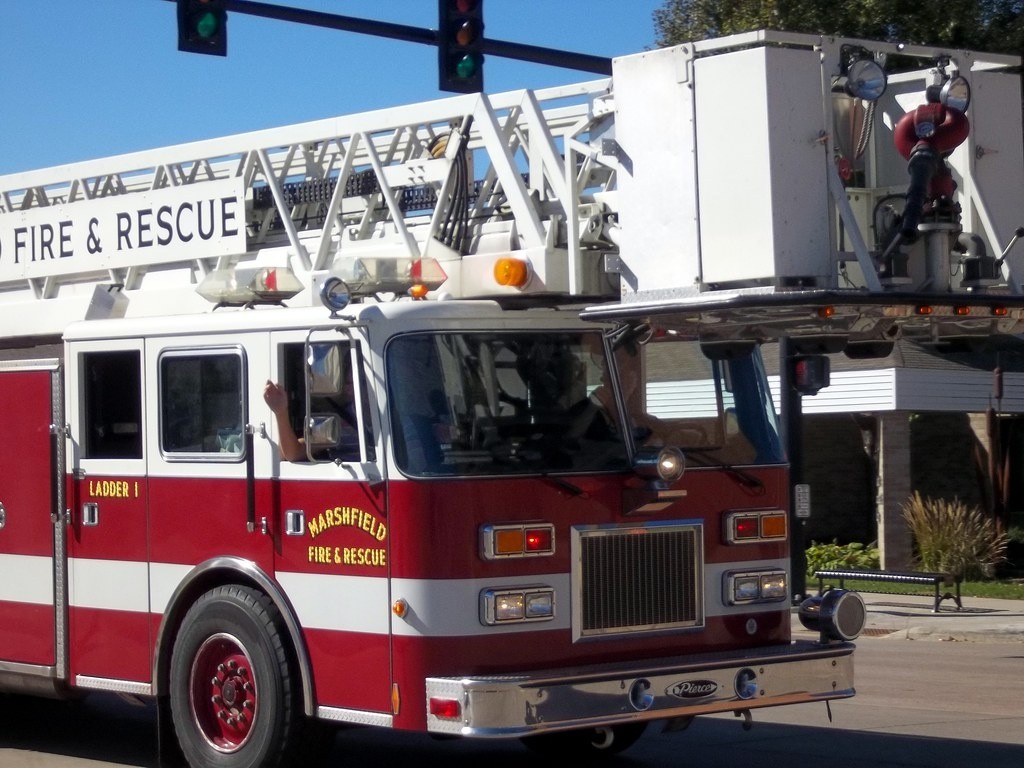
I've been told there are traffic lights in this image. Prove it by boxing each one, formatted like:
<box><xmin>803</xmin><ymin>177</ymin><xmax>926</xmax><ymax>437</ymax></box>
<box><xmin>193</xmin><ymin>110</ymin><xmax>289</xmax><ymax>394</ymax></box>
<box><xmin>437</xmin><ymin>0</ymin><xmax>488</xmax><ymax>93</ymax></box>
<box><xmin>177</xmin><ymin>0</ymin><xmax>229</xmax><ymax>57</ymax></box>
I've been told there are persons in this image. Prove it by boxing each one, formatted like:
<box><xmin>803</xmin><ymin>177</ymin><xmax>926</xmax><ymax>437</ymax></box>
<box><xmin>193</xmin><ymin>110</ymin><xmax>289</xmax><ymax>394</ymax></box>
<box><xmin>565</xmin><ymin>363</ymin><xmax>659</xmax><ymax>473</ymax></box>
<box><xmin>263</xmin><ymin>359</ymin><xmax>427</xmax><ymax>472</ymax></box>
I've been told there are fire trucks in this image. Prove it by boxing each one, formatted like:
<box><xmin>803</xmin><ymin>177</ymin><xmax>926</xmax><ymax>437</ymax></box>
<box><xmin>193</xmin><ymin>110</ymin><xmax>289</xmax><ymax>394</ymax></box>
<box><xmin>1</xmin><ymin>28</ymin><xmax>1024</xmax><ymax>768</ymax></box>
